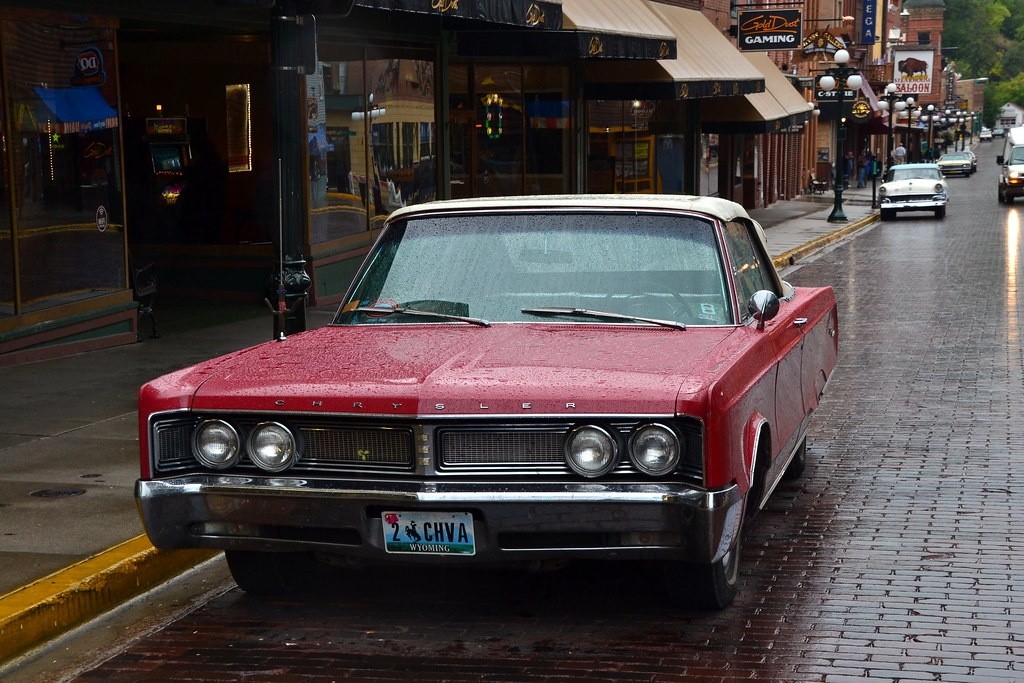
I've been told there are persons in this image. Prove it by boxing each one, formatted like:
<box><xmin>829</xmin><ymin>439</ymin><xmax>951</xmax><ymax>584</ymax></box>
<box><xmin>182</xmin><ymin>139</ymin><xmax>222</xmax><ymax>243</ymax></box>
<box><xmin>843</xmin><ymin>143</ymin><xmax>943</xmax><ymax>188</ymax></box>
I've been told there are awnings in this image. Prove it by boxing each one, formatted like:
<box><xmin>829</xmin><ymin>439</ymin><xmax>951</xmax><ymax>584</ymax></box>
<box><xmin>353</xmin><ymin>0</ymin><xmax>813</xmax><ymax>136</ymax></box>
<box><xmin>20</xmin><ymin>86</ymin><xmax>119</xmax><ymax>134</ymax></box>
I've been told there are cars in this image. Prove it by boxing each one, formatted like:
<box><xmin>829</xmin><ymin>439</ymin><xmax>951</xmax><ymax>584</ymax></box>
<box><xmin>130</xmin><ymin>191</ymin><xmax>842</xmax><ymax>613</ymax></box>
<box><xmin>877</xmin><ymin>162</ymin><xmax>949</xmax><ymax>220</ymax></box>
<box><xmin>936</xmin><ymin>152</ymin><xmax>973</xmax><ymax>178</ymax></box>
<box><xmin>963</xmin><ymin>150</ymin><xmax>978</xmax><ymax>171</ymax></box>
<box><xmin>992</xmin><ymin>129</ymin><xmax>1005</xmax><ymax>138</ymax></box>
<box><xmin>979</xmin><ymin>130</ymin><xmax>992</xmax><ymax>142</ymax></box>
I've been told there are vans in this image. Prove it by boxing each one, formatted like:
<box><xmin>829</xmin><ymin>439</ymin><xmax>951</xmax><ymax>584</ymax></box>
<box><xmin>996</xmin><ymin>126</ymin><xmax>1024</xmax><ymax>204</ymax></box>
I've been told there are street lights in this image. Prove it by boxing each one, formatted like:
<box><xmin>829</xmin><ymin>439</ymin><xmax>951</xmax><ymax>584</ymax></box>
<box><xmin>876</xmin><ymin>82</ymin><xmax>907</xmax><ymax>173</ymax></box>
<box><xmin>940</xmin><ymin>109</ymin><xmax>977</xmax><ymax>155</ymax></box>
<box><xmin>819</xmin><ymin>47</ymin><xmax>863</xmax><ymax>225</ymax></box>
<box><xmin>920</xmin><ymin>104</ymin><xmax>939</xmax><ymax>163</ymax></box>
<box><xmin>898</xmin><ymin>97</ymin><xmax>921</xmax><ymax>164</ymax></box>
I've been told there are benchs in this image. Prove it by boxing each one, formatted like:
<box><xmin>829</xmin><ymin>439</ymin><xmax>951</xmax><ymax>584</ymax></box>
<box><xmin>810</xmin><ymin>172</ymin><xmax>828</xmax><ymax>195</ymax></box>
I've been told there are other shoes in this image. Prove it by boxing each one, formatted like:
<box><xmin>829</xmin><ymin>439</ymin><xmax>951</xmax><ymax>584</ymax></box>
<box><xmin>857</xmin><ymin>185</ymin><xmax>865</xmax><ymax>188</ymax></box>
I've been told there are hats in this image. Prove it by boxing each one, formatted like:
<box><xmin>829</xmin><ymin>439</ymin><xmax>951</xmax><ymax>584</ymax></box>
<box><xmin>899</xmin><ymin>143</ymin><xmax>902</xmax><ymax>146</ymax></box>
